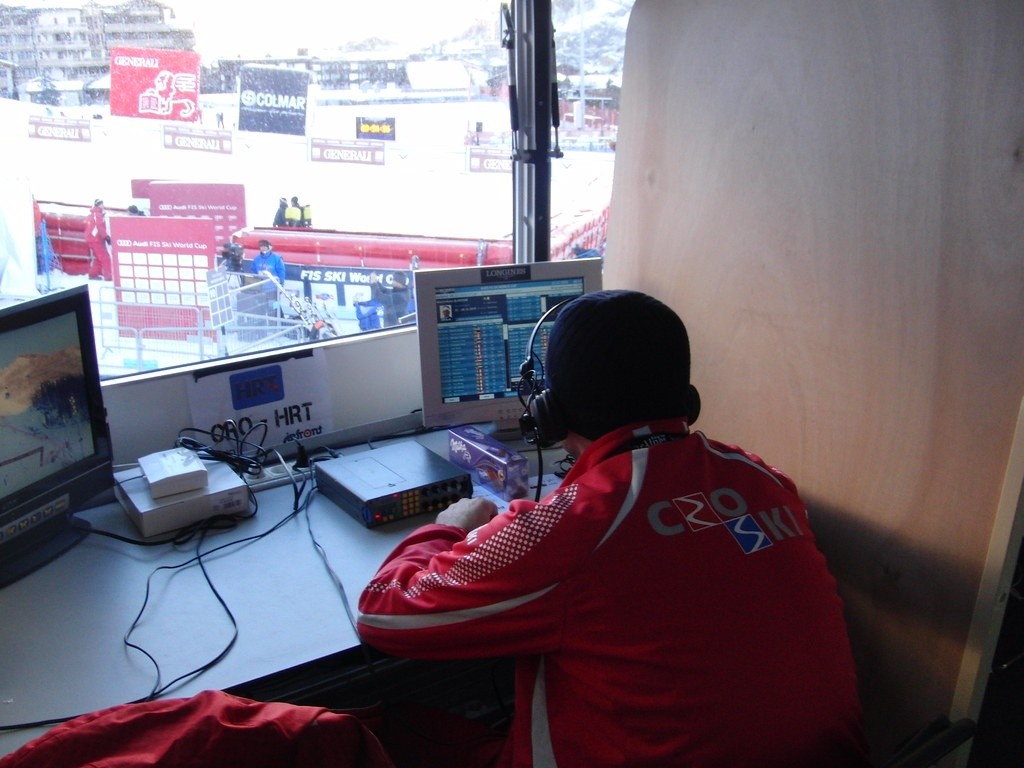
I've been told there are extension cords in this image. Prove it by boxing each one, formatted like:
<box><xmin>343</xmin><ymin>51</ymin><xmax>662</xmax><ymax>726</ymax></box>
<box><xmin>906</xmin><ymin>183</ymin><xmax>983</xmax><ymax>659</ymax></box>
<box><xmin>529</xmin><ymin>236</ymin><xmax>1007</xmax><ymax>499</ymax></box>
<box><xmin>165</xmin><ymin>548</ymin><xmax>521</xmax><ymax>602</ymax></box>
<box><xmin>244</xmin><ymin>460</ymin><xmax>317</xmax><ymax>493</ymax></box>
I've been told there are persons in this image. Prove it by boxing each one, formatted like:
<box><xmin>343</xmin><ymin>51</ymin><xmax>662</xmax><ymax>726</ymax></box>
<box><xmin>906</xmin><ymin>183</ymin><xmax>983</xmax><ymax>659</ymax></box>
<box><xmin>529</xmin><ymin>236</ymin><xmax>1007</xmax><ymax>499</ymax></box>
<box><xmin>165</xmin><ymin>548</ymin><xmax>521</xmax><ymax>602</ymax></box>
<box><xmin>273</xmin><ymin>197</ymin><xmax>312</xmax><ymax>227</ymax></box>
<box><xmin>440</xmin><ymin>305</ymin><xmax>453</xmax><ymax>321</ymax></box>
<box><xmin>84</xmin><ymin>199</ymin><xmax>111</xmax><ymax>280</ymax></box>
<box><xmin>250</xmin><ymin>240</ymin><xmax>290</xmax><ymax>338</ymax></box>
<box><xmin>357</xmin><ymin>290</ymin><xmax>859</xmax><ymax>768</ymax></box>
<box><xmin>370</xmin><ymin>271</ymin><xmax>410</xmax><ymax>327</ymax></box>
<box><xmin>127</xmin><ymin>206</ymin><xmax>146</xmax><ymax>216</ymax></box>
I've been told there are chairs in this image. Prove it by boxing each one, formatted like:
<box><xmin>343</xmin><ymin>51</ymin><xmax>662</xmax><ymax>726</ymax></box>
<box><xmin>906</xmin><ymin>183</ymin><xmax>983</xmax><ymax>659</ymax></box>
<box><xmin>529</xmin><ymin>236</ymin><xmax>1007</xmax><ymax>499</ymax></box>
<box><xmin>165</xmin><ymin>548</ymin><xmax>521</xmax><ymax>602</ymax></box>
<box><xmin>871</xmin><ymin>714</ymin><xmax>977</xmax><ymax>768</ymax></box>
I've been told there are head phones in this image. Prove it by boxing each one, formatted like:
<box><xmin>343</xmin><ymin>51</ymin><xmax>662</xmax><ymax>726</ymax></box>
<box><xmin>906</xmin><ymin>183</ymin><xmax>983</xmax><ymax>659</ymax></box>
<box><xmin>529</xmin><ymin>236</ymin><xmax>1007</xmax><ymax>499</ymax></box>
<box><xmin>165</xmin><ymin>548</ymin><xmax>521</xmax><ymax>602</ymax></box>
<box><xmin>519</xmin><ymin>295</ymin><xmax>701</xmax><ymax>451</ymax></box>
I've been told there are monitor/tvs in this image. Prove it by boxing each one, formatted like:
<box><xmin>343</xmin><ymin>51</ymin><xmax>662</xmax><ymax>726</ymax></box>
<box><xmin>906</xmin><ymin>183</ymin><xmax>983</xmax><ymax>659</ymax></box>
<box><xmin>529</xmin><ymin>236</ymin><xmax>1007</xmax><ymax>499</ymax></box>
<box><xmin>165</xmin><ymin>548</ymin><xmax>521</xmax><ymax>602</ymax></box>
<box><xmin>414</xmin><ymin>256</ymin><xmax>603</xmax><ymax>450</ymax></box>
<box><xmin>0</xmin><ymin>284</ymin><xmax>115</xmax><ymax>589</ymax></box>
